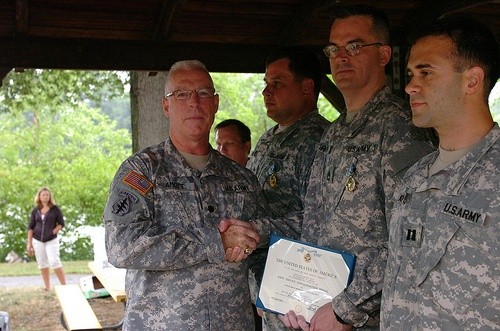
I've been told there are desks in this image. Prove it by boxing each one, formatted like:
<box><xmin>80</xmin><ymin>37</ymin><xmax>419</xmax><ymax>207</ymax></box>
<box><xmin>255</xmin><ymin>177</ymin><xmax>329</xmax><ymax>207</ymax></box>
<box><xmin>87</xmin><ymin>260</ymin><xmax>127</xmax><ymax>331</ymax></box>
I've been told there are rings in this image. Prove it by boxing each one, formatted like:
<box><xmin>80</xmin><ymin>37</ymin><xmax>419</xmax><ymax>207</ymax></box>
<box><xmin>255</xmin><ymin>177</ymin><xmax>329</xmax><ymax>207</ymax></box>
<box><xmin>244</xmin><ymin>247</ymin><xmax>251</xmax><ymax>254</ymax></box>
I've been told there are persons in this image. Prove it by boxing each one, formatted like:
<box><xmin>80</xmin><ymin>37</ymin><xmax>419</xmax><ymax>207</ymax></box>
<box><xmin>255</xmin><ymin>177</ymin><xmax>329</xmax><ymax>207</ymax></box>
<box><xmin>212</xmin><ymin>5</ymin><xmax>438</xmax><ymax>331</ymax></box>
<box><xmin>102</xmin><ymin>60</ymin><xmax>275</xmax><ymax>331</ymax></box>
<box><xmin>378</xmin><ymin>21</ymin><xmax>500</xmax><ymax>331</ymax></box>
<box><xmin>27</xmin><ymin>186</ymin><xmax>66</xmax><ymax>291</ymax></box>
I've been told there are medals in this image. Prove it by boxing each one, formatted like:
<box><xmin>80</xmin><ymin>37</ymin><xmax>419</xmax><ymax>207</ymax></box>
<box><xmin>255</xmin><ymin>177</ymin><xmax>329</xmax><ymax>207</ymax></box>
<box><xmin>269</xmin><ymin>175</ymin><xmax>276</xmax><ymax>188</ymax></box>
<box><xmin>345</xmin><ymin>174</ymin><xmax>356</xmax><ymax>191</ymax></box>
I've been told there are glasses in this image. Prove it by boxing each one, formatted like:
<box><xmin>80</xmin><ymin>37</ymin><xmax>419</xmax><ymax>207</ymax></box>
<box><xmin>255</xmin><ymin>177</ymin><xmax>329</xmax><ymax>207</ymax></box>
<box><xmin>322</xmin><ymin>39</ymin><xmax>383</xmax><ymax>58</ymax></box>
<box><xmin>165</xmin><ymin>87</ymin><xmax>215</xmax><ymax>99</ymax></box>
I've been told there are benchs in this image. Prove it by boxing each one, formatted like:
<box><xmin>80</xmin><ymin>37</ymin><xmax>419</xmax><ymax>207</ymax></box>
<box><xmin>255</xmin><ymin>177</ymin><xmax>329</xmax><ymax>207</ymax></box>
<box><xmin>53</xmin><ymin>284</ymin><xmax>103</xmax><ymax>331</ymax></box>
